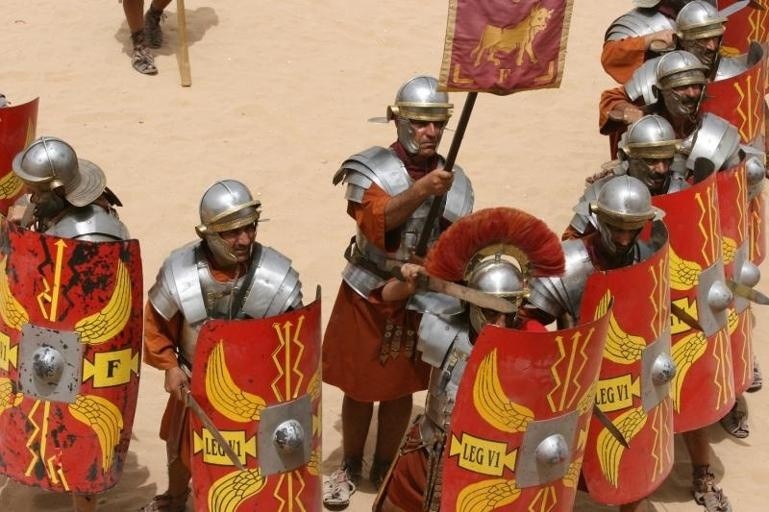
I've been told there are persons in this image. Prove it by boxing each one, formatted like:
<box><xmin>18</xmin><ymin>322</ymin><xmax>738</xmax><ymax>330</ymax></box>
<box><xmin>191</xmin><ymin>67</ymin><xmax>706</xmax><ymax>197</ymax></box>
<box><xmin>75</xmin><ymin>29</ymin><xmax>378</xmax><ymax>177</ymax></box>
<box><xmin>0</xmin><ymin>136</ymin><xmax>144</xmax><ymax>511</ymax></box>
<box><xmin>143</xmin><ymin>178</ymin><xmax>325</xmax><ymax>512</ymax></box>
<box><xmin>523</xmin><ymin>1</ymin><xmax>768</xmax><ymax>512</ymax></box>
<box><xmin>325</xmin><ymin>75</ymin><xmax>475</xmax><ymax>507</ymax></box>
<box><xmin>119</xmin><ymin>0</ymin><xmax>180</xmax><ymax>77</ymax></box>
<box><xmin>0</xmin><ymin>87</ymin><xmax>40</xmax><ymax>220</ymax></box>
<box><xmin>371</xmin><ymin>207</ymin><xmax>567</xmax><ymax>512</ymax></box>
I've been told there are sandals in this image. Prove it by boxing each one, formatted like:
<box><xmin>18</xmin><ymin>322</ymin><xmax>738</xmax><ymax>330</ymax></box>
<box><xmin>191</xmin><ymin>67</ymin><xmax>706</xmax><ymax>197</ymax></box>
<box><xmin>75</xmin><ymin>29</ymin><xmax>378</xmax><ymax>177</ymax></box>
<box><xmin>692</xmin><ymin>464</ymin><xmax>732</xmax><ymax>512</ymax></box>
<box><xmin>746</xmin><ymin>352</ymin><xmax>763</xmax><ymax>392</ymax></box>
<box><xmin>323</xmin><ymin>457</ymin><xmax>367</xmax><ymax>508</ymax></box>
<box><xmin>719</xmin><ymin>397</ymin><xmax>750</xmax><ymax>438</ymax></box>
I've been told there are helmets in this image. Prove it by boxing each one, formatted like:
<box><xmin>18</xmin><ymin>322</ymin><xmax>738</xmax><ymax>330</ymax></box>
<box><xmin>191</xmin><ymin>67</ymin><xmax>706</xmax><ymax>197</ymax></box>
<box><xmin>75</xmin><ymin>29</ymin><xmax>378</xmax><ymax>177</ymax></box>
<box><xmin>195</xmin><ymin>180</ymin><xmax>263</xmax><ymax>266</ymax></box>
<box><xmin>387</xmin><ymin>74</ymin><xmax>454</xmax><ymax>158</ymax></box>
<box><xmin>589</xmin><ymin>175</ymin><xmax>658</xmax><ymax>258</ymax></box>
<box><xmin>622</xmin><ymin>114</ymin><xmax>682</xmax><ymax>191</ymax></box>
<box><xmin>467</xmin><ymin>253</ymin><xmax>531</xmax><ymax>336</ymax></box>
<box><xmin>652</xmin><ymin>0</ymin><xmax>729</xmax><ymax>124</ymax></box>
<box><xmin>11</xmin><ymin>137</ymin><xmax>107</xmax><ymax>219</ymax></box>
